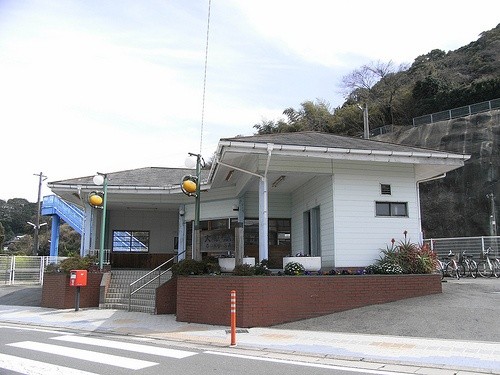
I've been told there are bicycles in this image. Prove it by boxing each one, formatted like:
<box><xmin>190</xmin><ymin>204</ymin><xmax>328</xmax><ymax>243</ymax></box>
<box><xmin>436</xmin><ymin>250</ymin><xmax>478</xmax><ymax>280</ymax></box>
<box><xmin>477</xmin><ymin>247</ymin><xmax>500</xmax><ymax>278</ymax></box>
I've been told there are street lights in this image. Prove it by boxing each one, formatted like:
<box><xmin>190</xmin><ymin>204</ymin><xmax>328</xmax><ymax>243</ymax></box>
<box><xmin>181</xmin><ymin>153</ymin><xmax>201</xmax><ymax>261</ymax></box>
<box><xmin>87</xmin><ymin>172</ymin><xmax>108</xmax><ymax>270</ymax></box>
